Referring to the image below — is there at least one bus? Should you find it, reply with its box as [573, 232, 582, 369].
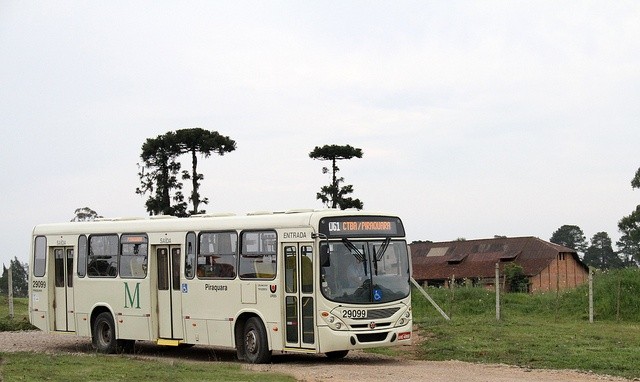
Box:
[27, 207, 413, 364]
